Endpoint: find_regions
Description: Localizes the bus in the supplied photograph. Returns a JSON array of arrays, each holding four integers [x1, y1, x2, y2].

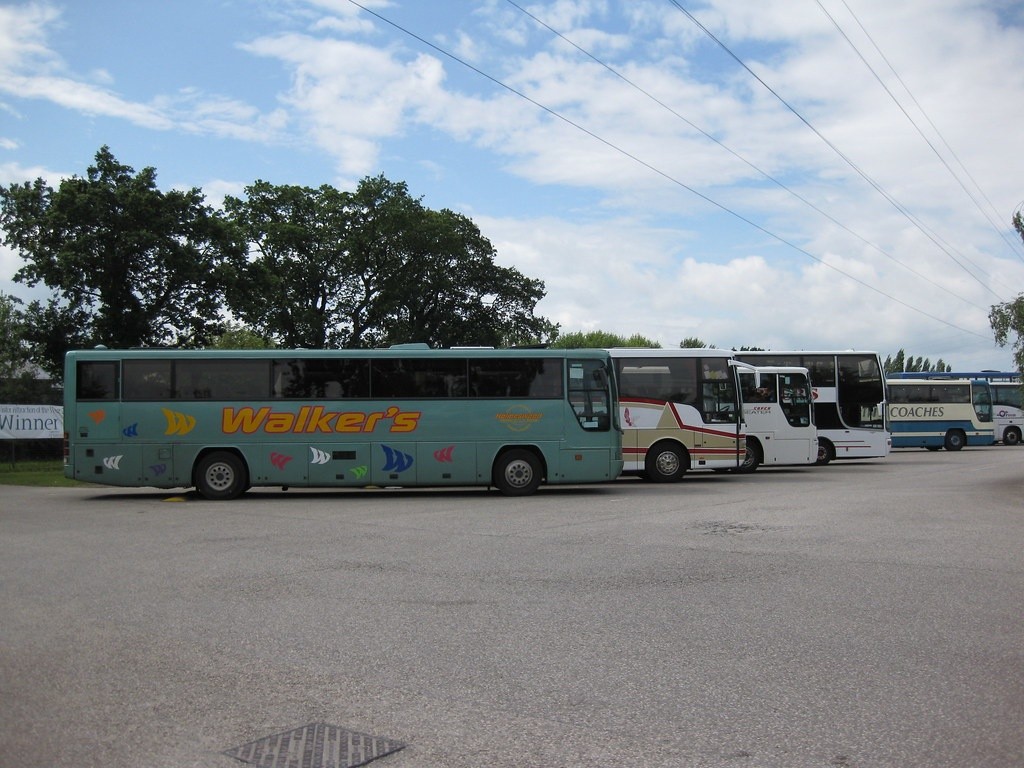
[[566, 348, 757, 483], [623, 365, 819, 474], [733, 350, 892, 465], [886, 370, 1024, 445], [61, 343, 625, 500], [884, 379, 996, 451]]
